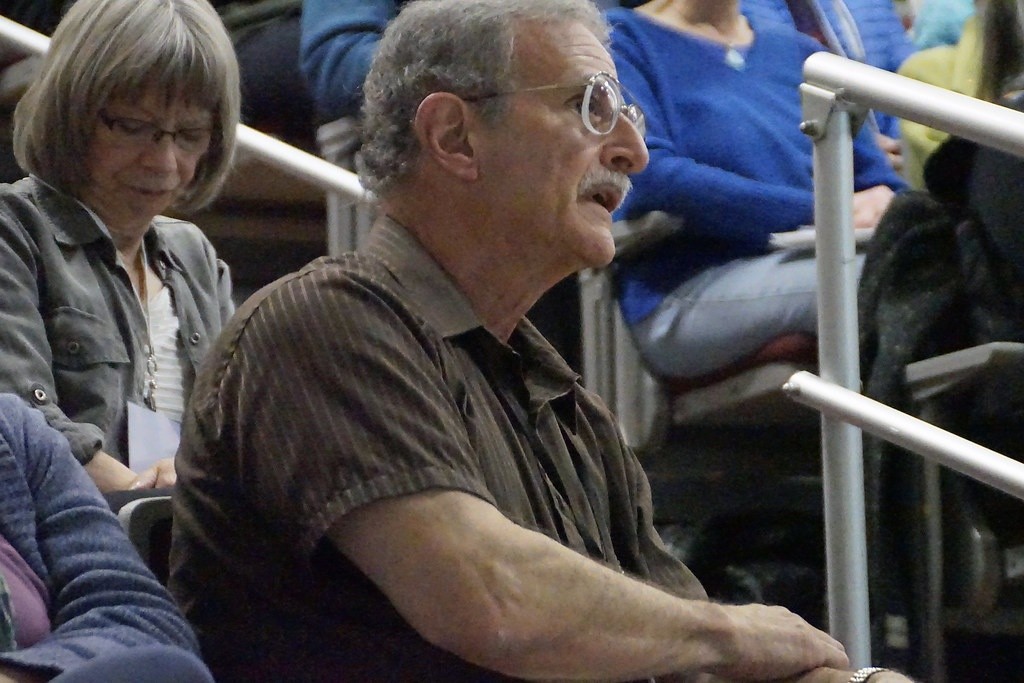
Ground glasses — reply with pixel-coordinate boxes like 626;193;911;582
98;108;222;154
397;71;646;142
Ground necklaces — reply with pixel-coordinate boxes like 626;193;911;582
698;19;749;69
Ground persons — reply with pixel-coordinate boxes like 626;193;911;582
169;1;1024;683
2;385;214;682
0;2;246;579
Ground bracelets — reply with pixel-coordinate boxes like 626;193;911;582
849;664;888;682
